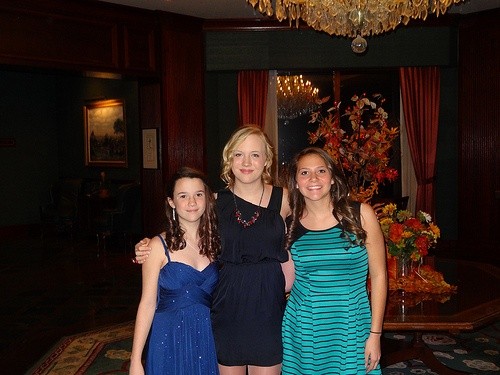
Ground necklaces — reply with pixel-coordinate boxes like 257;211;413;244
183;235;200;255
233;184;264;228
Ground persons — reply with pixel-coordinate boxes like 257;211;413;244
129;167;220;375
280;147;389;375
135;125;380;375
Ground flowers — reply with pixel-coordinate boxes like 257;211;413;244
380;204;440;264
307;89;402;207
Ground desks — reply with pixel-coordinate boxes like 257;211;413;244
380;290;500;331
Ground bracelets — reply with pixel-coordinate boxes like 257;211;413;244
370;330;382;335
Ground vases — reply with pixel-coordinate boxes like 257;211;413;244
397;259;411;296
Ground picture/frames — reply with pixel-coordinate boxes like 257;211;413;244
82;98;129;169
